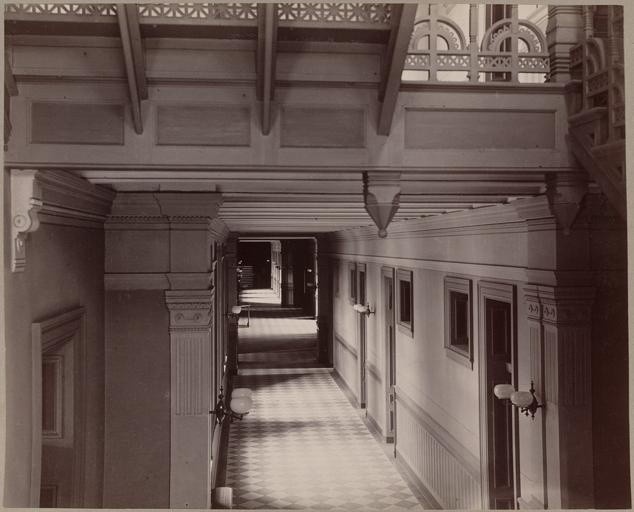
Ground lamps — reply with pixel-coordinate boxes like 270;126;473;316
493;379;545;421
209;384;254;426
353;303;375;317
225;306;242;321
